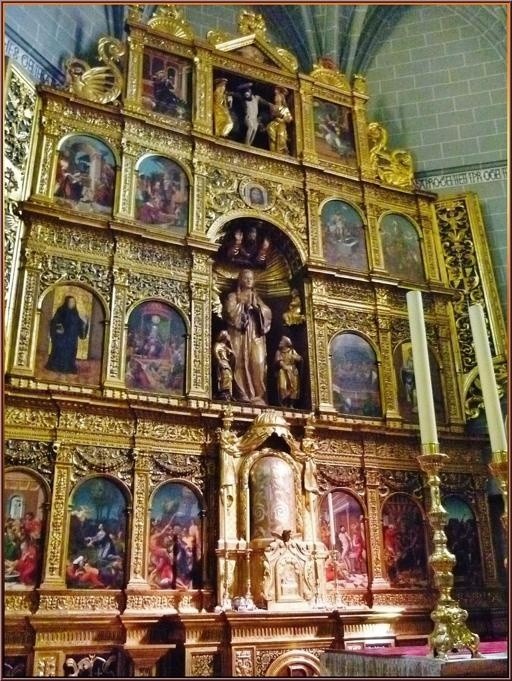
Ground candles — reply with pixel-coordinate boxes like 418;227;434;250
406;291;441;456
467;305;509;464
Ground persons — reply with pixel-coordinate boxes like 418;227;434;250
214;226;303;406
6;506;200;592
213;76;292;155
323;517;478;585
